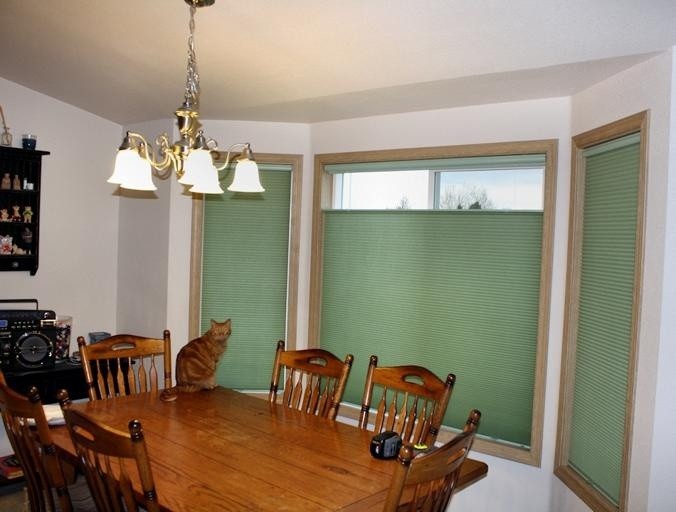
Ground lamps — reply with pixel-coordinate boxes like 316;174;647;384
105;0;265;197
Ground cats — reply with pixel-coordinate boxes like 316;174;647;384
159;318;232;401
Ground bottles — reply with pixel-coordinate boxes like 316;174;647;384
396;441;429;466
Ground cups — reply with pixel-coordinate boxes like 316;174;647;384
21;135;37;150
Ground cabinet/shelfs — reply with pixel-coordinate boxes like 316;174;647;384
0;147;50;276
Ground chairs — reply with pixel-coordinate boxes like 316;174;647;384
57;389;159;512
357;355;455;446
76;330;172;401
0;373;74;512
267;340;354;419
383;409;481;512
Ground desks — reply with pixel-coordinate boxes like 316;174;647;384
27;384;487;512
0;358;135;494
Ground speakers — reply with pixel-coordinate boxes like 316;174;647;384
0;299;55;373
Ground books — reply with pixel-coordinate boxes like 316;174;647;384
0;453;25;481
